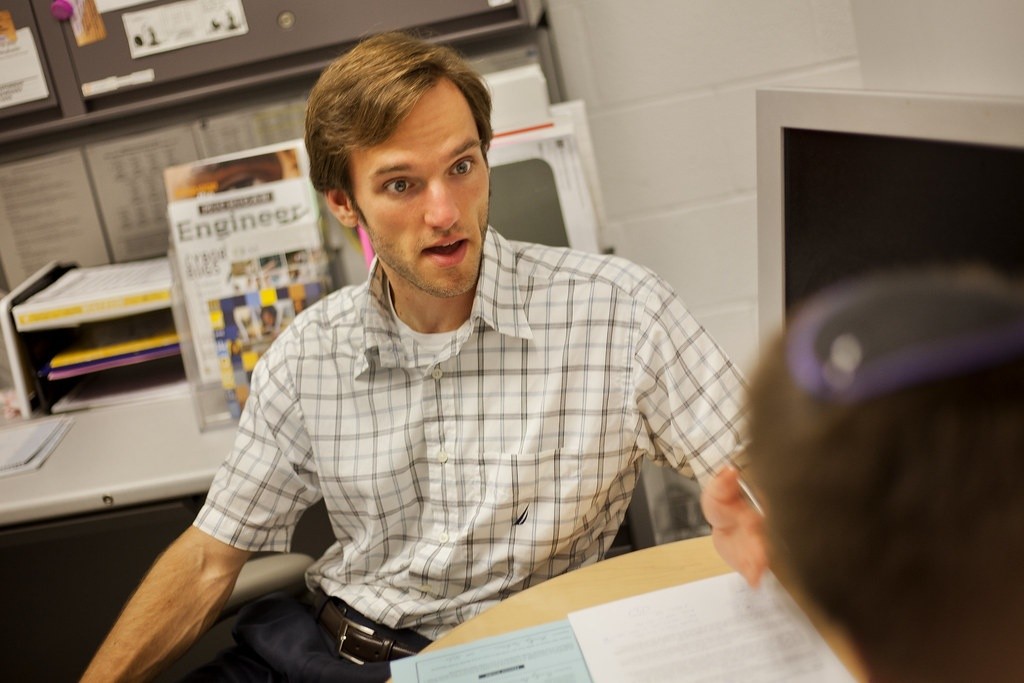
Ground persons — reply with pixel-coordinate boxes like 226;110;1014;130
743;270;1024;683
80;32;770;682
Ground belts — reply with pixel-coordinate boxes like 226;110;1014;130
304;587;420;665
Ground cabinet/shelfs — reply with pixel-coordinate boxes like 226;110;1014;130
0;387;671;682
0;0;567;146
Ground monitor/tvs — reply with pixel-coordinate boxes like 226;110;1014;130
755;86;1023;362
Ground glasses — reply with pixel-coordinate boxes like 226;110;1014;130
793;260;1024;411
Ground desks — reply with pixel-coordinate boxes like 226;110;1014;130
418;536;870;683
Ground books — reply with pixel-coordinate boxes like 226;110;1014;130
479;63;608;255
164;137;339;416
1;260;190;478
